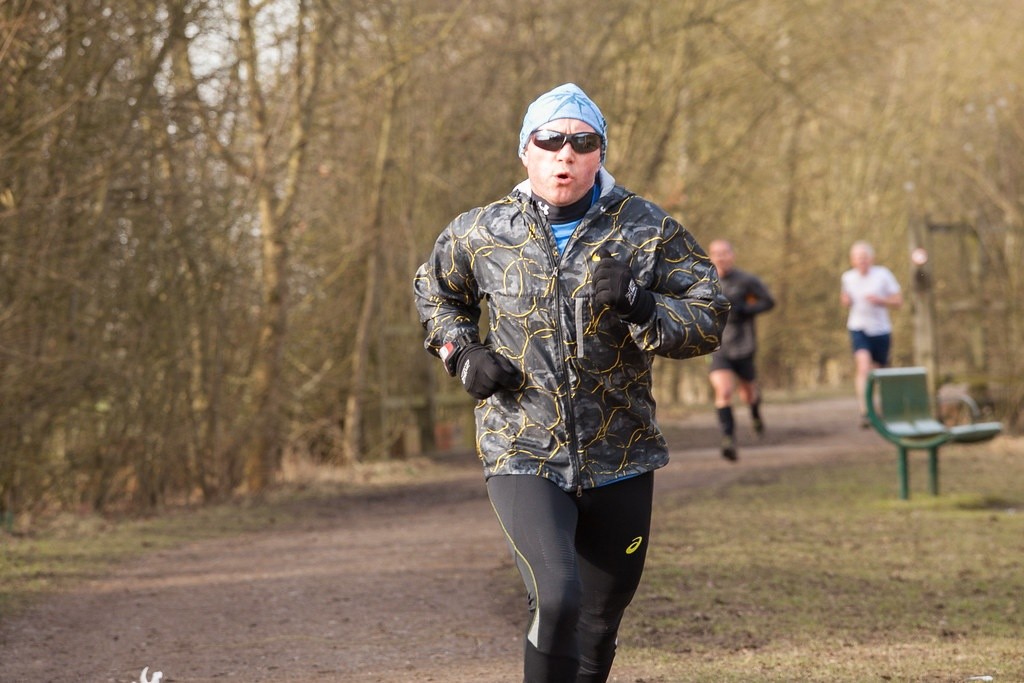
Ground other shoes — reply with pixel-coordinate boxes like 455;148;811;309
722;435;737;462
750;412;767;439
860;412;873;426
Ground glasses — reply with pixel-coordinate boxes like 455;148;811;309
530;128;602;154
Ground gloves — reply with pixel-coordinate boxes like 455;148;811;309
728;304;743;325
591;250;658;328
455;347;523;399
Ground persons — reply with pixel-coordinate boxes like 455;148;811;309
841;242;903;428
414;83;728;682
707;239;775;460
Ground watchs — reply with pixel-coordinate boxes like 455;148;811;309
438;333;478;376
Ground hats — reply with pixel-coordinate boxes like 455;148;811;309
518;82;607;170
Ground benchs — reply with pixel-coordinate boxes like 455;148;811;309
864;365;1005;500
